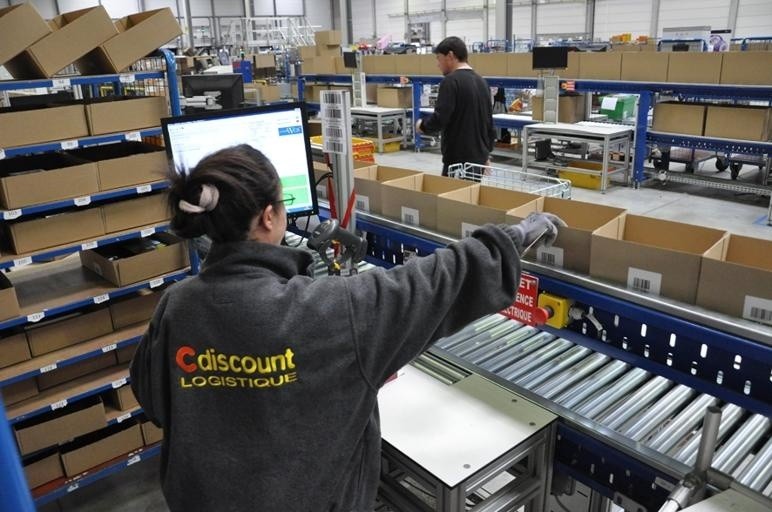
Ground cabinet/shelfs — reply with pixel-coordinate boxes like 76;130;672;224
0;2;199;512
296;71;771;204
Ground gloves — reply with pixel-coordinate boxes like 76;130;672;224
514;210;570;248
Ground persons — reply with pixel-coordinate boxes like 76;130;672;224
128;145;568;512
415;36;497;182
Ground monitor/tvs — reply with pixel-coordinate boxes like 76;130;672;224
161;100;319;245
343;52;357;68
181;72;245;114
533;46;568;78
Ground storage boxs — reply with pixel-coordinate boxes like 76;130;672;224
364;50;534;76
253;31;342;76
568;50;771;85
312;149;772;325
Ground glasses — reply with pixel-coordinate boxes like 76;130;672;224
273;193;296;206
